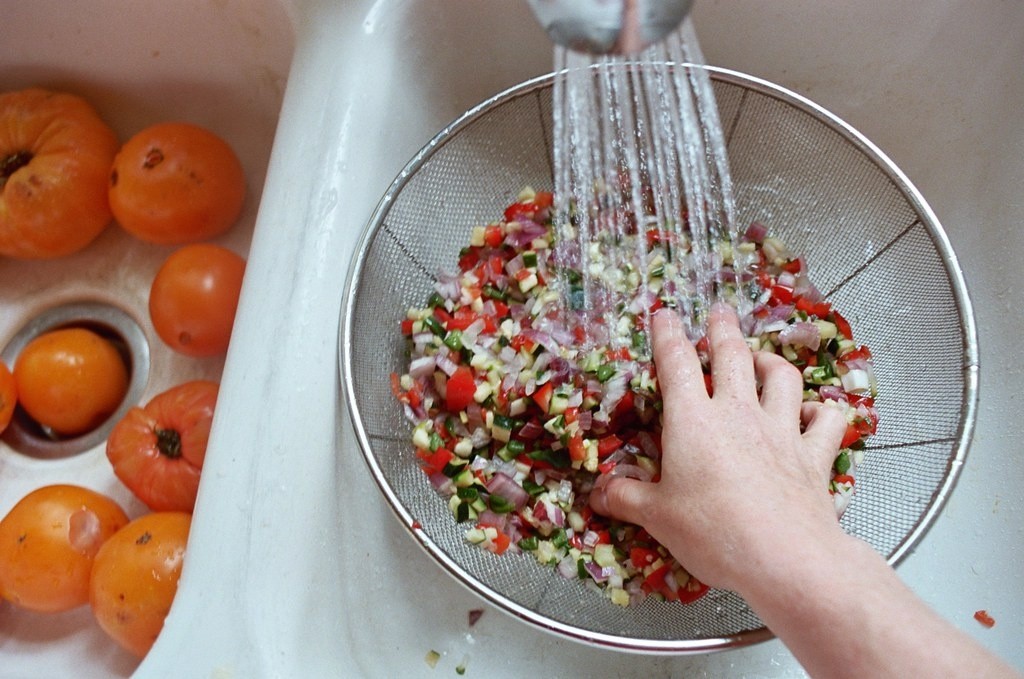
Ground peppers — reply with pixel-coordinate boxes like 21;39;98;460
398;191;876;605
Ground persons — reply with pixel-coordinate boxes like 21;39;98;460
589;301;1024;679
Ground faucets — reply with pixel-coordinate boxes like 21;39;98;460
528;2;695;57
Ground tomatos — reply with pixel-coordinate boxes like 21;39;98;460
1;86;246;659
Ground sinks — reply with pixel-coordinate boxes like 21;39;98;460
2;1;296;679
326;2;1024;679
